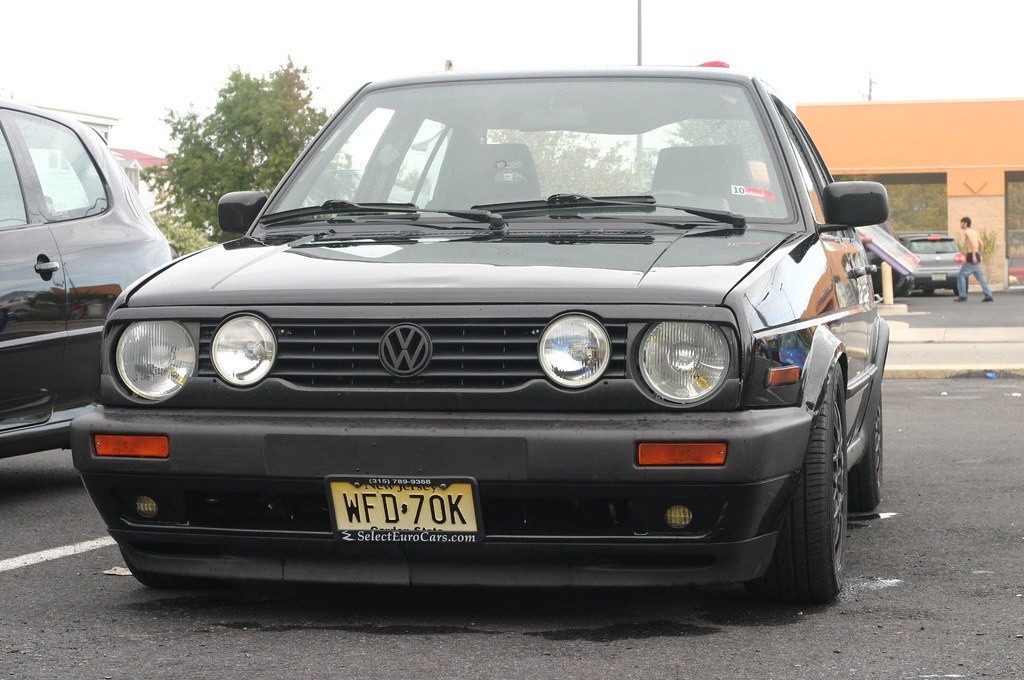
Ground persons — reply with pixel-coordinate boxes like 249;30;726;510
954;217;993;302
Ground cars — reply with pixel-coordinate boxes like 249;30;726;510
70;64;890;614
850;212;969;299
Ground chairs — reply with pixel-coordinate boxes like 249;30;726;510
427;140;543;212
650;141;753;208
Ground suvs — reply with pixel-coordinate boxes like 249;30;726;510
0;100;178;459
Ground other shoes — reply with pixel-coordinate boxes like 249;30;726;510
953;296;967;302
981;297;993;302
